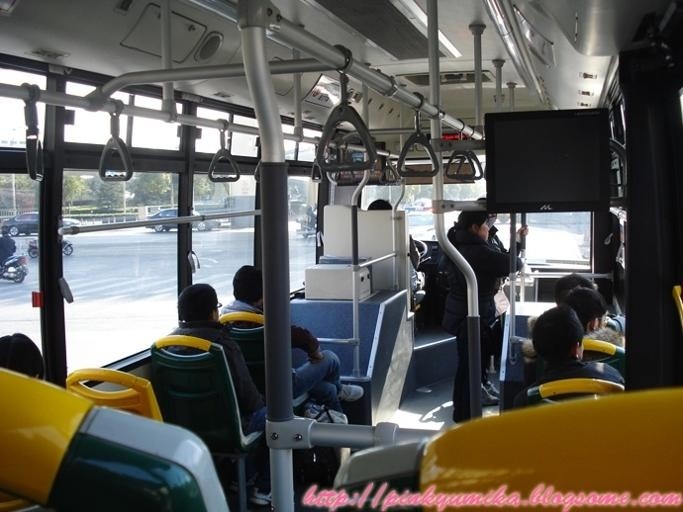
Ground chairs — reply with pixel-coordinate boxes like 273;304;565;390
331;386;683;512
147;335;265;511
66;367;161;420
0;368;231;511
510;376;626;406
216;312;309;407
582;339;624;376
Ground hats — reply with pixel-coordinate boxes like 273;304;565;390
233;266;263;301
177;285;218;322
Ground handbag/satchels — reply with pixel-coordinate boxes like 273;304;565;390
304;401;347;424
494;290;509;317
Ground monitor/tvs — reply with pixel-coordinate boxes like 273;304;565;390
485;107;610;213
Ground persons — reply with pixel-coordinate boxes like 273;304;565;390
367;199;425;298
0;230;17;267
507;304;624;410
448;212;522;424
508;287;626;377
473;197;526;405
162;283;271;505
219;265;363;423
543;275;610;303
0;332;46;380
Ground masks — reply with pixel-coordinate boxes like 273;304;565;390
486;216;496;231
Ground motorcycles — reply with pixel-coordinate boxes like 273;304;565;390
0;251;30;284
295;198;320;242
28;236;75;260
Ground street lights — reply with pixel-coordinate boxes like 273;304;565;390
4;120;24;219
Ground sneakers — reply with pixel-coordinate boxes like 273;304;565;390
487;382;499;397
338;384;363;402
248;487;271;505
480;384;500;405
230;471;259;494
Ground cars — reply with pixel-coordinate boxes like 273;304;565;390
400;205;441;243
0;209;83;236
144;206;215;236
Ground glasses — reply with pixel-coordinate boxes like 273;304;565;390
215;303;222;309
595;317;609;327
